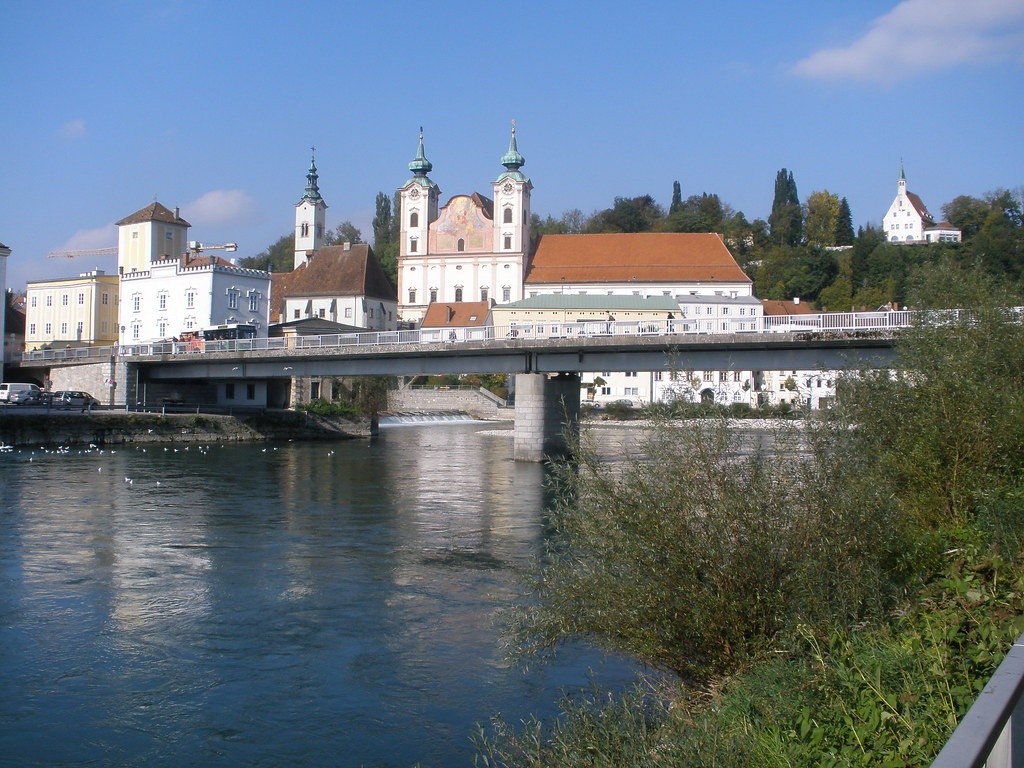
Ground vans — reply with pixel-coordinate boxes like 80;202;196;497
0;382;42;403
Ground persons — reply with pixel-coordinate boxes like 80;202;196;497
81;395;90;413
605;311;611;336
667;312;677;335
449;329;456;342
173;334;223;354
113;339;118;356
505;330;519;339
33;347;37;351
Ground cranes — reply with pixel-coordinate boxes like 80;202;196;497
47;241;238;263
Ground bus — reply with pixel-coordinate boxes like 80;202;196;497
204;324;257;351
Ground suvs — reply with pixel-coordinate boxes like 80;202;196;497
51;390;99;411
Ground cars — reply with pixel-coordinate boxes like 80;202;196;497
9;388;55;405
